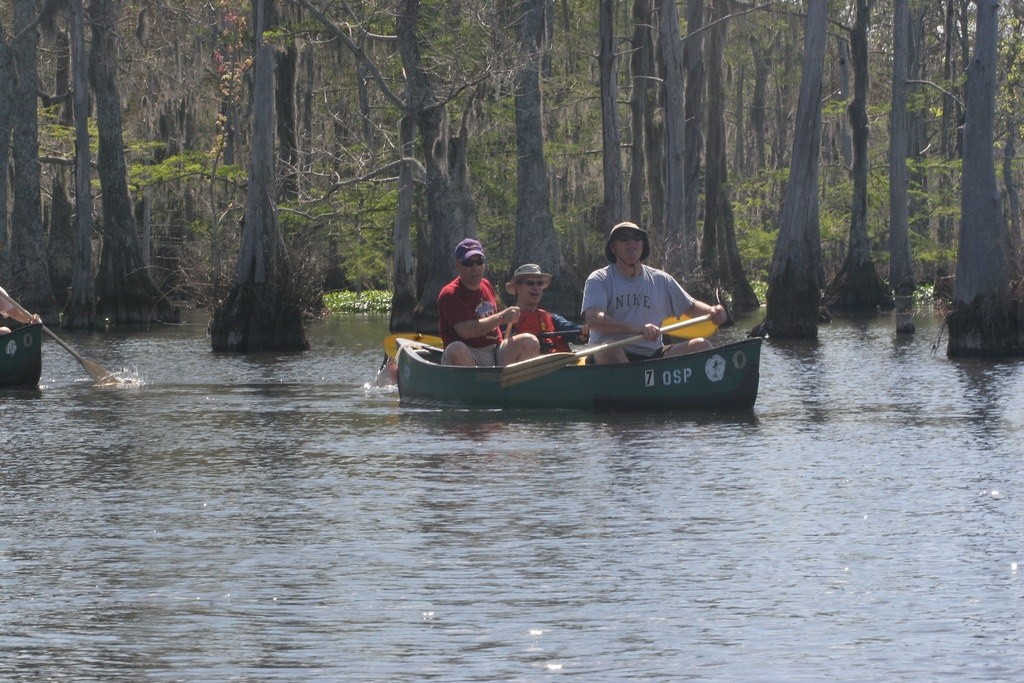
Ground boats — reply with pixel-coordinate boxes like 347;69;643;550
394;336;762;412
0;322;44;388
382;307;718;385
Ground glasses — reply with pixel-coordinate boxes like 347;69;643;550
618;234;642;242
462;258;484;266
525;280;544;286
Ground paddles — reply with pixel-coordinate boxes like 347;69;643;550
383;327;583;359
498;313;712;388
0;288;120;384
504;309;517;339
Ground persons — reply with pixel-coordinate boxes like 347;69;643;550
0;286;41;335
505;264;590;354
437;238;540;367
581;222;727;365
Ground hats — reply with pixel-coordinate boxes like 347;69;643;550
506;264;552;296
454;239;483;263
605;222;650;263
